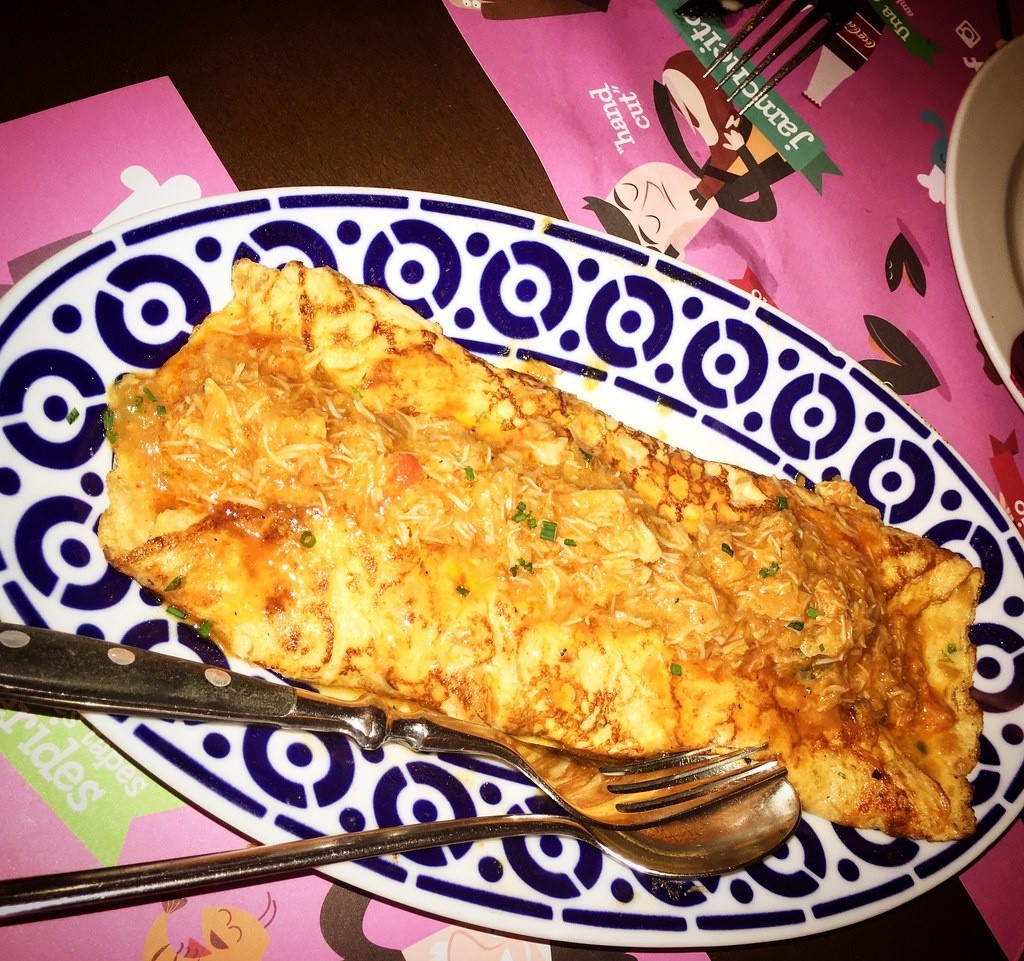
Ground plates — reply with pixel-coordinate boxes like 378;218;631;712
0;186;1024;951
942;34;1024;414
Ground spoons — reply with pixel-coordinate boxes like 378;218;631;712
0;777;800;931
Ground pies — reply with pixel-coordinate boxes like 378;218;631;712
95;257;985;842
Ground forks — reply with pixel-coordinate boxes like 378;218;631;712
701;0;862;118
0;622;789;829
